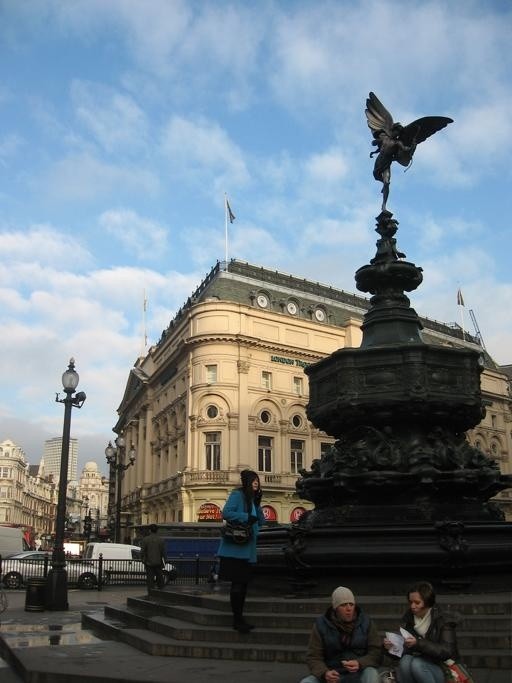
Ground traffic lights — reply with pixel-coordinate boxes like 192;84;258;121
83;524;89;536
85;516;89;522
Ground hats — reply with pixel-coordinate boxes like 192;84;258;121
241;470;257;487
332;586;355;610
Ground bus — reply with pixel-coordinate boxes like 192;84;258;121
131;523;290;578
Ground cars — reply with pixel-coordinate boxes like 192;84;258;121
1;531;107;590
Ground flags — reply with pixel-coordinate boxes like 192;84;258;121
226;196;236;224
457;287;465;308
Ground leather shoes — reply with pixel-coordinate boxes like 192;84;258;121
233;616;256;634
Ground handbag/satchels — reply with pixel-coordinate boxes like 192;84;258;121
221;525;253;544
439;659;477;683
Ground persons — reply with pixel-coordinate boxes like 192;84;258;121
384;582;460;683
216;470;265;635
141;524;168;595
370;122;417;211
299;586;382;683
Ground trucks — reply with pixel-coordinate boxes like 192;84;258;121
0;522;39;558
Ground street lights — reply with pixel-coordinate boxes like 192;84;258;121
105;436;136;543
48;355;86;612
101;476;112;514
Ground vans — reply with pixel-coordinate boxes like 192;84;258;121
83;542;177;585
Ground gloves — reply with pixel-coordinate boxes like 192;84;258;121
249;515;257;524
